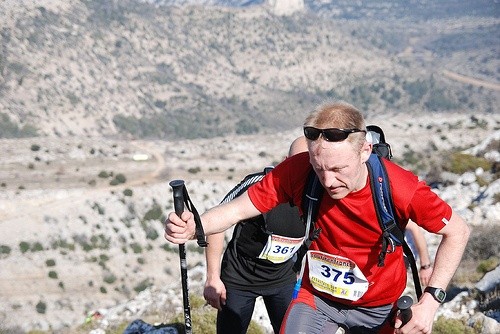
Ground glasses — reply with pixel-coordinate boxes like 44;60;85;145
304;127;371;141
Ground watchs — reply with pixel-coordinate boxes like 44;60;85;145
420;263;432;271
423;286;447;304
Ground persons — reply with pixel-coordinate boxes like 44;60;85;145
162;96;472;334
201;131;314;333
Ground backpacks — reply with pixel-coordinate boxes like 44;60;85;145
298;125;424;304
